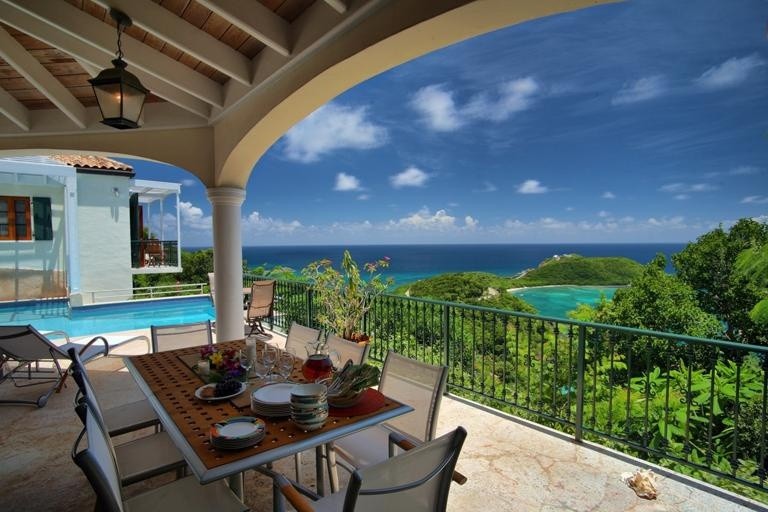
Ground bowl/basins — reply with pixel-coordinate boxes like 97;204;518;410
314;372;368;407
290;383;329;432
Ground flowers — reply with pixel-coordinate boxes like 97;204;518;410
197;343;250;379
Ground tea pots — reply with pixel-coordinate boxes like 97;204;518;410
301;341;342;382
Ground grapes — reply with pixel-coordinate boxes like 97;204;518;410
214;375;241;398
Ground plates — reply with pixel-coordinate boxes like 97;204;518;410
194;381;247;402
209;416;266;449
250;381;295;419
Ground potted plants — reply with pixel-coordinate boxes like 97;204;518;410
299;246;393;362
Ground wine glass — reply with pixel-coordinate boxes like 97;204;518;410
238;340;297;383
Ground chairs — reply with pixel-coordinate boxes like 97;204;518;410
244;279;279;339
283;319;325;361
270;425;467;511
330;350;452;473
68;402;249;511
317;333;371;374
149;318;213;355
0;324;110;407
67;345;165;450
205;272;216;330
67;369;192;487
147;237;164;265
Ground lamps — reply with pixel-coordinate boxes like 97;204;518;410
86;7;152;130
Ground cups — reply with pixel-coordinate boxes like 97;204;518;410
245;337;257;359
197;359;210;375
